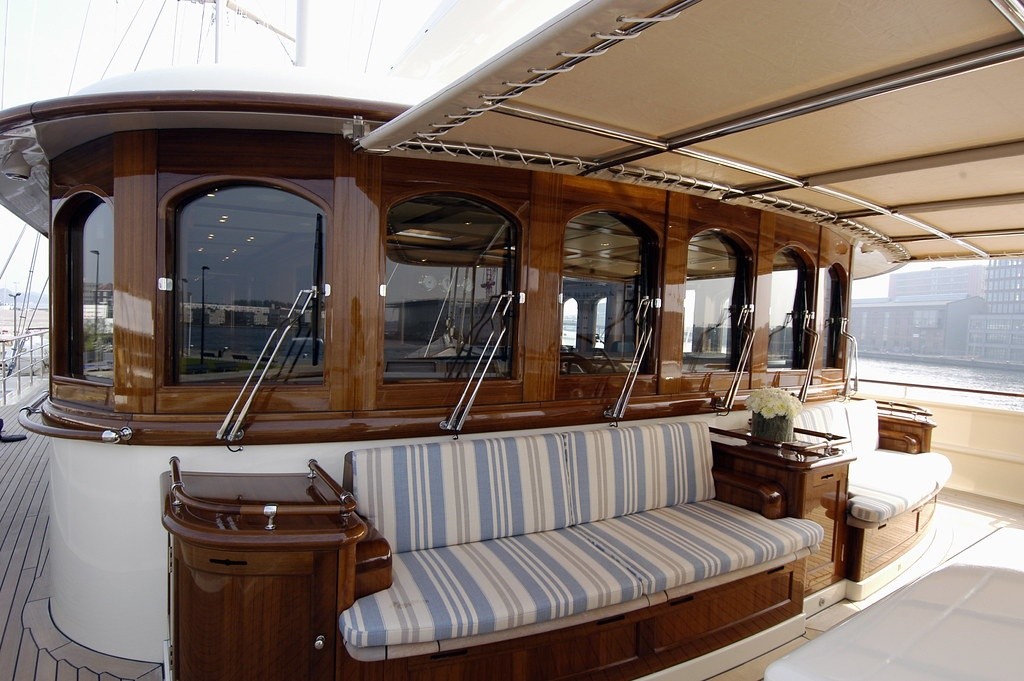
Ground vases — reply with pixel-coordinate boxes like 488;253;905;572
751;411;794;442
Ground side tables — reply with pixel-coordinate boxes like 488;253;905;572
851;397;937;453
709;419;857;600
158;456;368;681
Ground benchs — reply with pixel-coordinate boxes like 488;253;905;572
339;421;825;681
795;398;951;601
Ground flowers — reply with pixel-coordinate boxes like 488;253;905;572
744;386;804;420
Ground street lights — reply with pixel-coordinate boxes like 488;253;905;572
181;277;188;357
201;266;210;365
9;293;21;337
90;250;99;338
